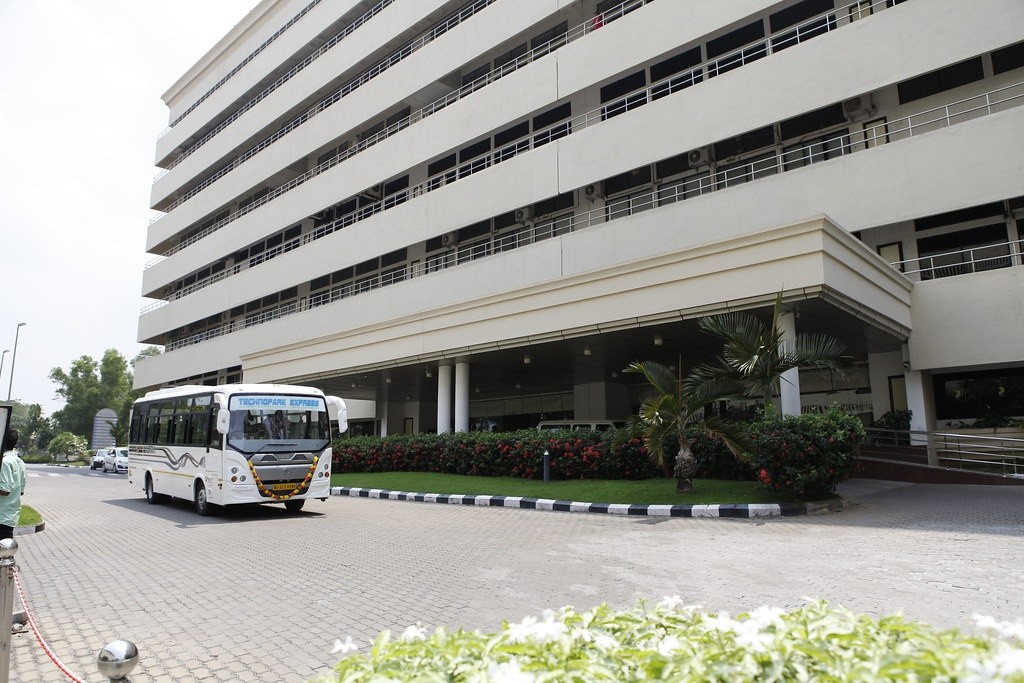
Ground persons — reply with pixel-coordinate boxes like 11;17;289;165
473;416;496;432
0;429;27;541
194;331;200;343
265;410;287;439
592;12;603;30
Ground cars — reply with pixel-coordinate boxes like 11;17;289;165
102;447;128;474
90;449;108;470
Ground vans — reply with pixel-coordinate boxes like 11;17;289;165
537;418;627;433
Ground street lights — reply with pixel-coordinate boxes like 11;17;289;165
0;349;10;380
7;323;27;401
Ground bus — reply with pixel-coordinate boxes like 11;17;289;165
127;384;348;517
